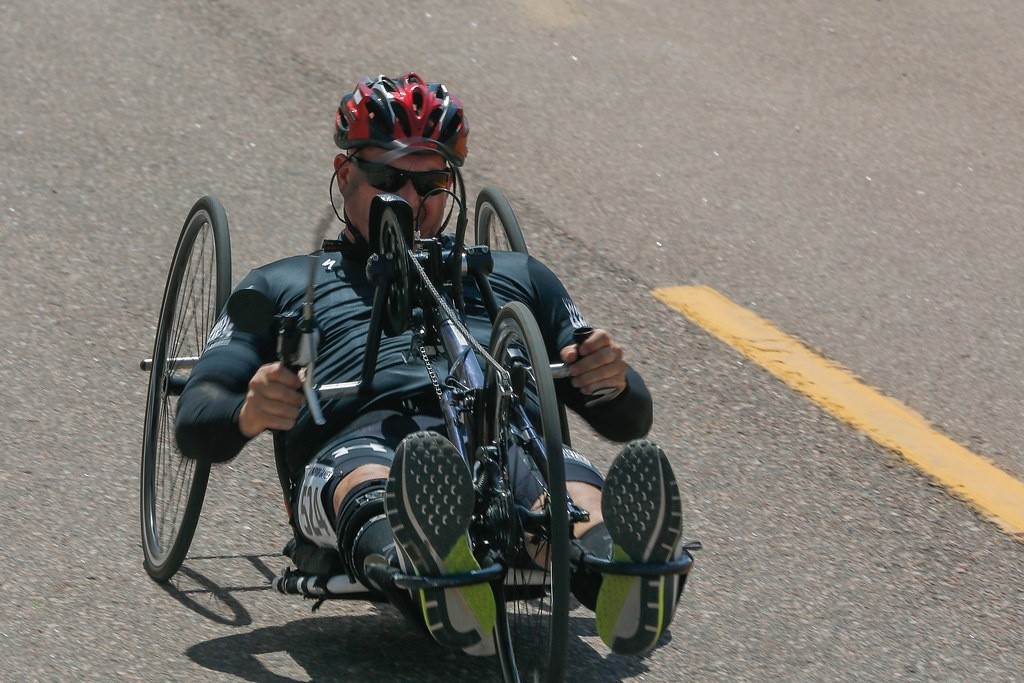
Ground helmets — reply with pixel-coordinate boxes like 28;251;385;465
332;71;469;167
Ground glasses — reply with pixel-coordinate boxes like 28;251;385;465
347;154;453;196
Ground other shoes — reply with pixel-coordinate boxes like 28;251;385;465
384;431;496;649
596;440;684;655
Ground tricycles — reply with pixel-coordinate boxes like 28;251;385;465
140;188;702;683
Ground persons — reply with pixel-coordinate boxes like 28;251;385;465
175;71;684;657
